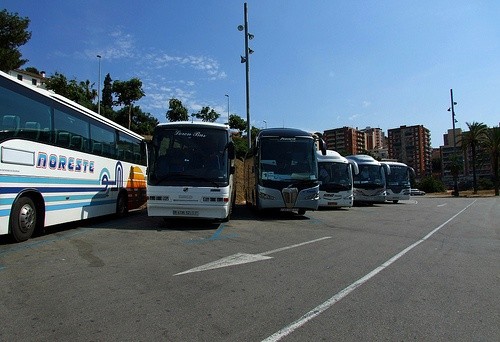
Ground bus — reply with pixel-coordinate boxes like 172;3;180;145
253;128;327;216
0;71;150;243
317;149;359;210
345;154;391;207
146;117;237;223
380;161;416;204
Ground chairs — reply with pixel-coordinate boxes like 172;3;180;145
0;115;141;163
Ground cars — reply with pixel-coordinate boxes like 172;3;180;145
411;189;426;196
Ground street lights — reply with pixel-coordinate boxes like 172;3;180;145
447;88;458;190
238;3;254;149
96;54;101;115
225;94;230;125
